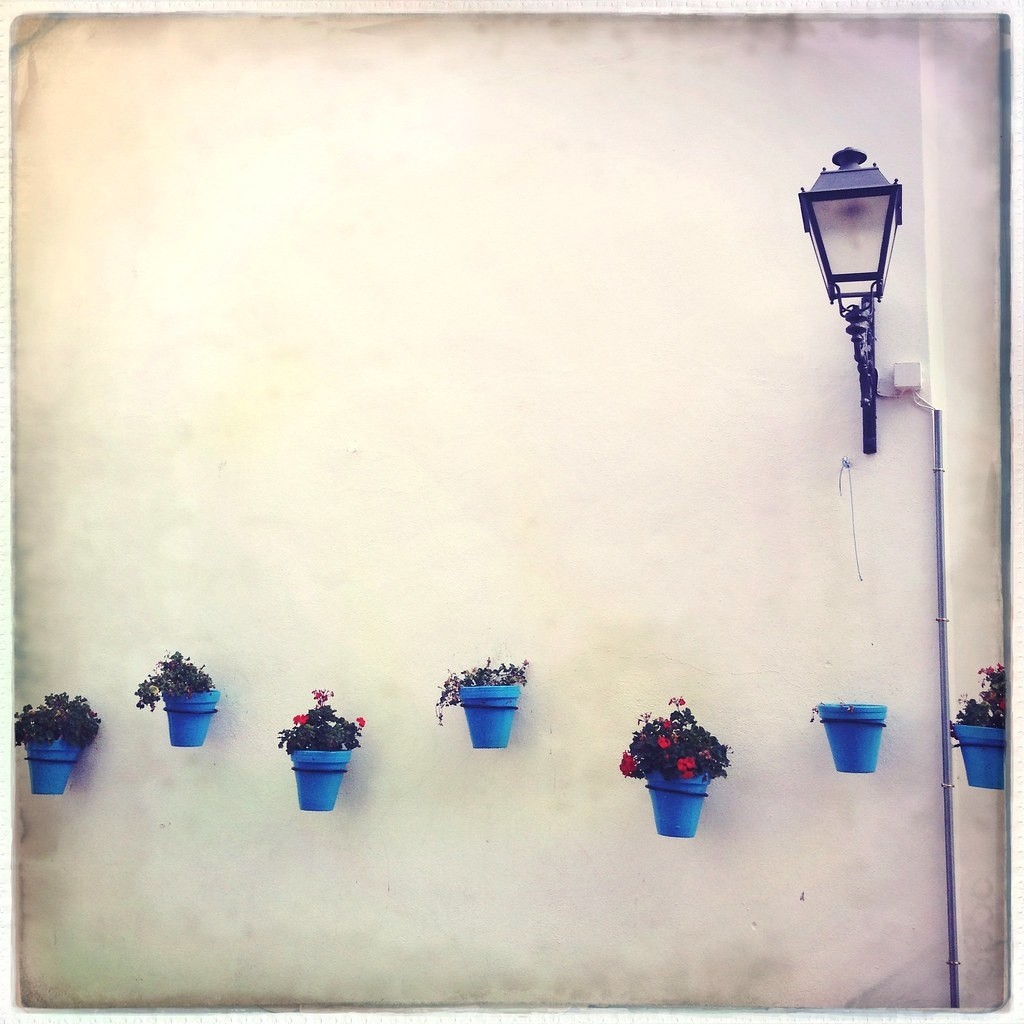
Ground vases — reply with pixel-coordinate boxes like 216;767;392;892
24;739;83;795
290;751;353;812
643;770;710;837
810;702;887;774
160;690;220;748
953;724;1005;790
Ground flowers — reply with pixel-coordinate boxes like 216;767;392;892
618;696;734;781
276;690;366;754
134;649;216;712
14;692;102;747
949;663;1007;737
435;657;530;726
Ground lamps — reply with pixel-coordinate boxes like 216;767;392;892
796;147;902;456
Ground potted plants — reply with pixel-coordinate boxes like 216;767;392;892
459;684;521;749
